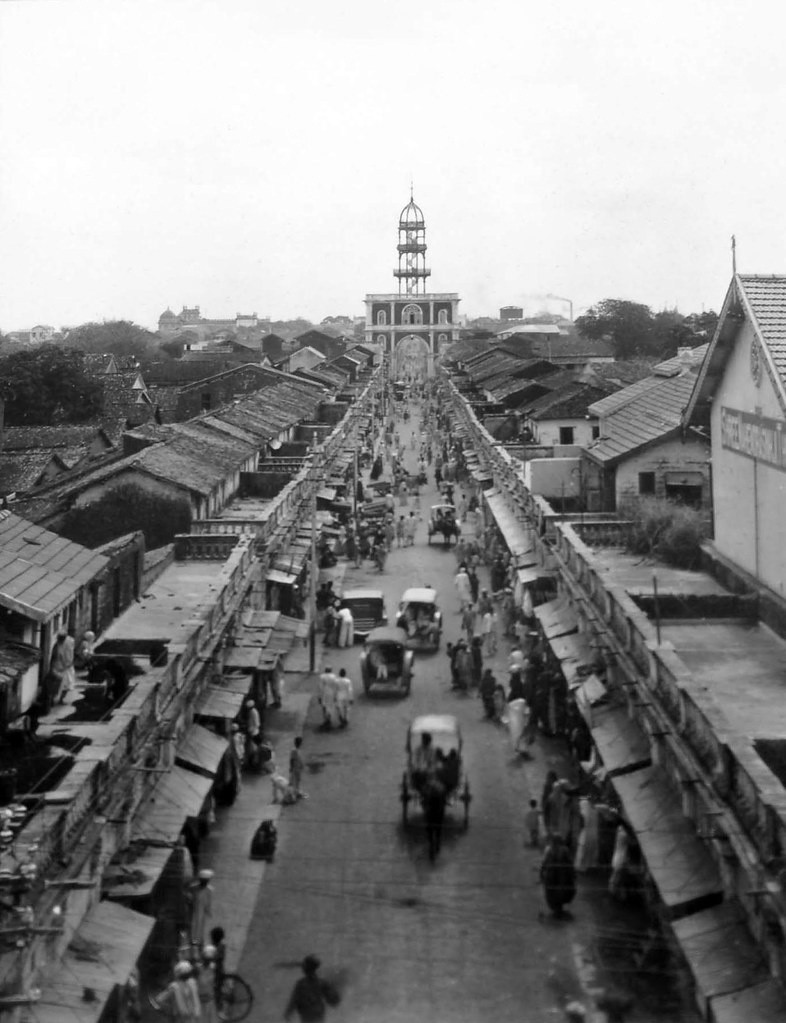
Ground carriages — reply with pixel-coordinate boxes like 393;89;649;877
396;583;445;649
428;505;460;547
400;713;473;867
360;615;414;698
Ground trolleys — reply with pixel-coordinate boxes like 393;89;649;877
375;485;397;495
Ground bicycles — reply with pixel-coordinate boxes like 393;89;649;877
146;941;254;1022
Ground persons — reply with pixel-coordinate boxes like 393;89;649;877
47;358;654;1023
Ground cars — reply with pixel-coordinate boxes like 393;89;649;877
336;590;388;636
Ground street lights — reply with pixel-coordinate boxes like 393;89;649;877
546;294;573;321
